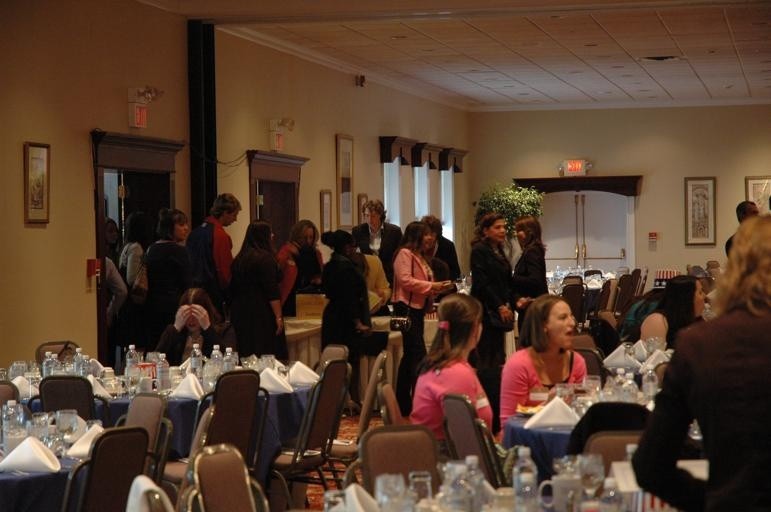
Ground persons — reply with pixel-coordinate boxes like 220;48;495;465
631;213;771;512
725;200;758;257
640;274;705;389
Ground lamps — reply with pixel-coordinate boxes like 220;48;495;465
130;85;164;103
266;115;298;134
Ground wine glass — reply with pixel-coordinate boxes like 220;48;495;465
553;453;606;503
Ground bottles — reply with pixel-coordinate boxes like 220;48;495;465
2;398;20;436
546;263;594;289
616;363;658;399
597;476;628;512
42;341;238;395
513;443;538;510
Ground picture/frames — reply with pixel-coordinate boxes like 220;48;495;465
320;190;332;244
358;193;369;226
24;142;52;226
683;176;717;246
744;175;770;218
335;133;354;231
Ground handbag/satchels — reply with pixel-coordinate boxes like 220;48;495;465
390;316;411;333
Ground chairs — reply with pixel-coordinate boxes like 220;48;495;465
1;266;728;510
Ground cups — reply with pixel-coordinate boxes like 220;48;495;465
0;357;41;398
85;419;103;433
555;371;602;413
625;444;638;459
30;411;66;457
375;456;488;511
241;352;277;373
536;475;581;512
